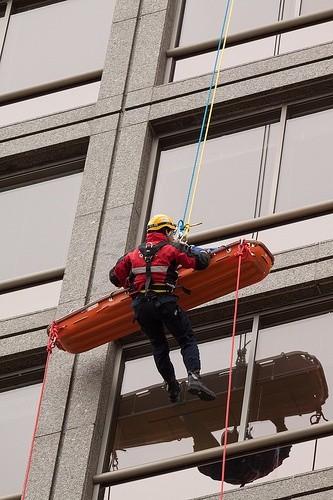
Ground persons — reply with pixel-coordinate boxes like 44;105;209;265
191;416;294;486
108;214;218;402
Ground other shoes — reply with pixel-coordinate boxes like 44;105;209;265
168;379;185;403
187;374;216;401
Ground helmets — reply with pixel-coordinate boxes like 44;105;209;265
147;214;177;232
